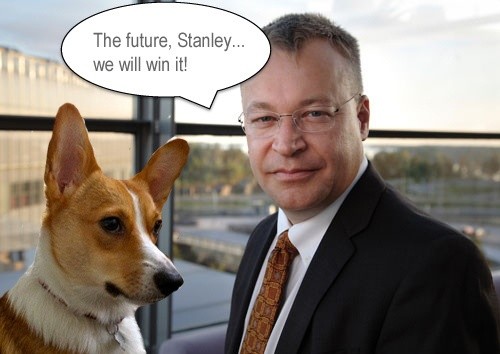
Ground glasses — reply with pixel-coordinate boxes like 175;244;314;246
237;88;364;138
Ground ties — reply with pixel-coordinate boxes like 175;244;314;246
240;229;300;354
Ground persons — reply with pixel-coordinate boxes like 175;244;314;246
223;11;499;354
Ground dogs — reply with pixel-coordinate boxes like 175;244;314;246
0;102;191;350
